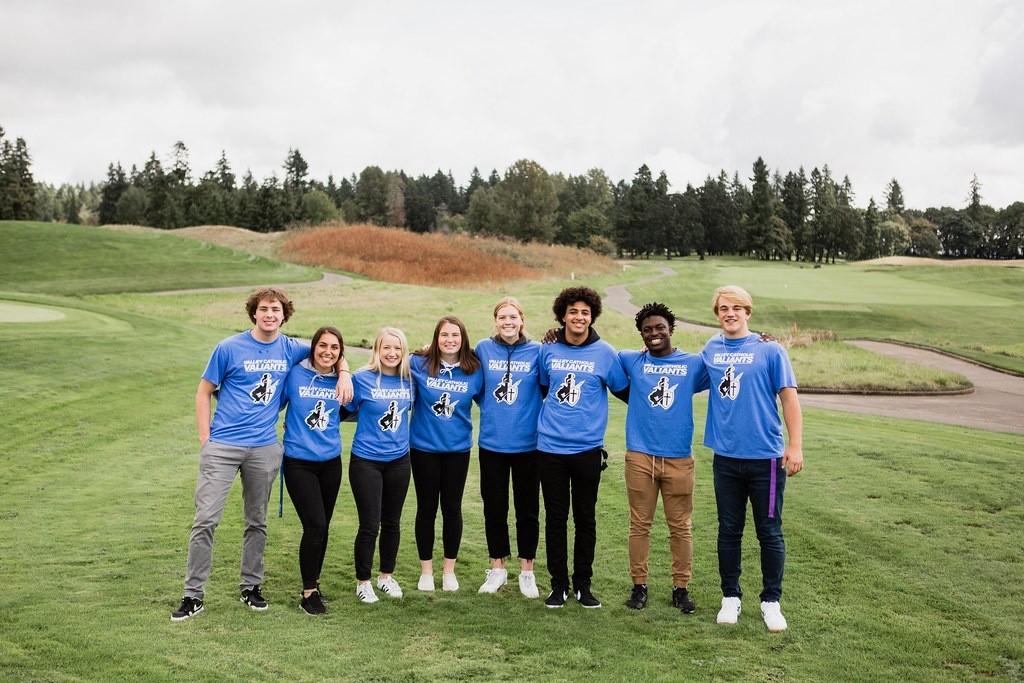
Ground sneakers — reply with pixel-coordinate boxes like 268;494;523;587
715;597;741;624
376;576;403;598
418;575;434;591
761;601;787;631
572;584;602;608
170;596;205;621
623;584;648;611
478;568;508;593
356;580;379;603
519;574;540;598
299;588;329;616
672;586;696;614
240;585;269;611
543;584;571;607
443;573;460;592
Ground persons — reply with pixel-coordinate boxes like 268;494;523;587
283;326;415;603
212;326;343;617
407;294;543;599
536;285;803;633
170;286;354;621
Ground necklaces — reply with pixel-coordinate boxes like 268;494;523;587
722;333;751;367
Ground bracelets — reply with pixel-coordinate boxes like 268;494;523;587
339;370;351;376
